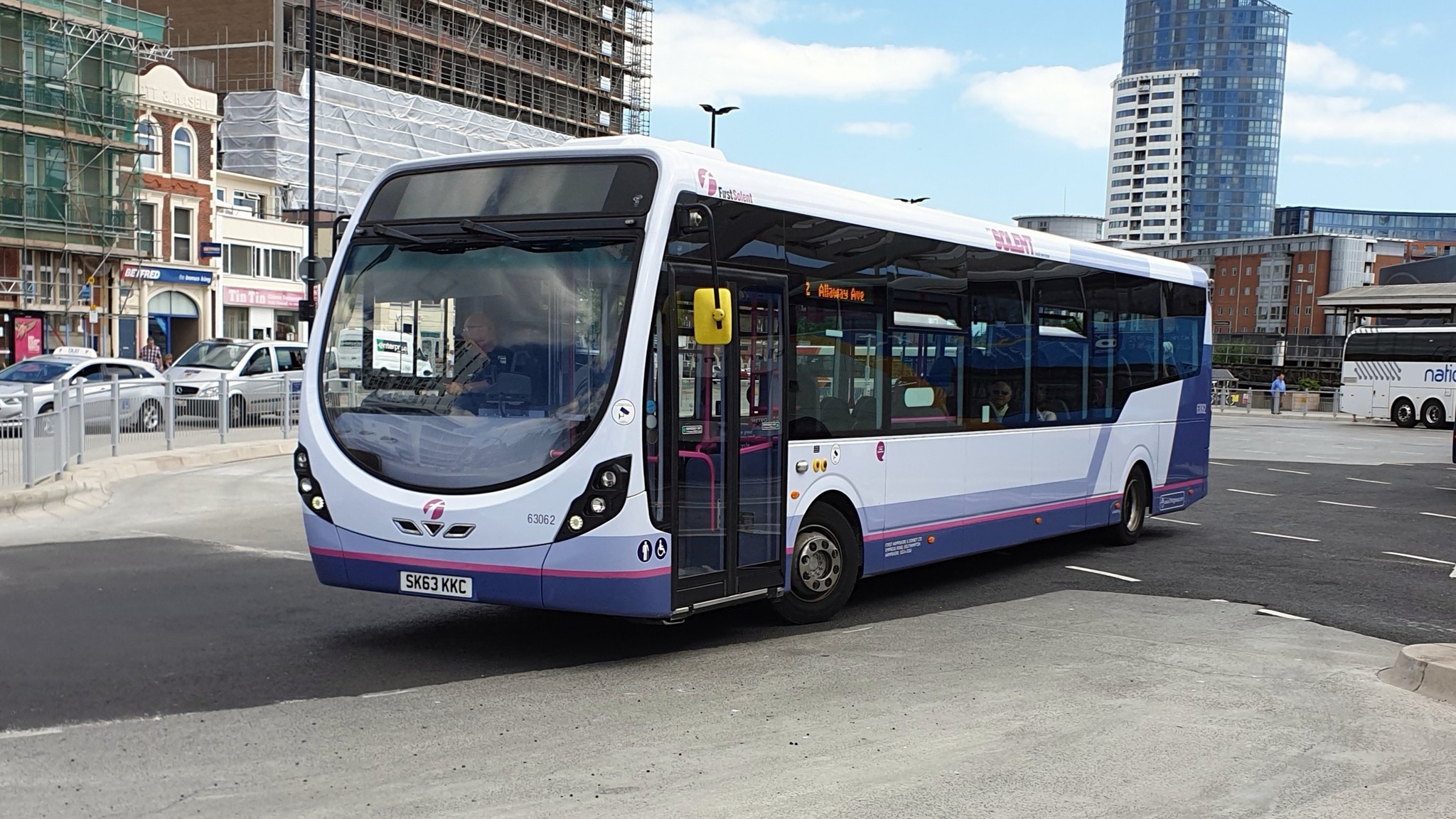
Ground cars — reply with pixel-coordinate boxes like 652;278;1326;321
0;346;169;437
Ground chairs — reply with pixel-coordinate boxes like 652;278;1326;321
821;341;1183;436
290;352;301;366
249;350;261;366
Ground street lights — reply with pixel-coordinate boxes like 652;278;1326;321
334;153;352;251
1281;252;1295;374
699;104;741;148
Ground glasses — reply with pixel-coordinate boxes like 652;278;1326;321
993;390;1010;396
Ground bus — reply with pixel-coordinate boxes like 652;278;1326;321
291;132;1214;627
772;344;987;408
1337;325;1456;430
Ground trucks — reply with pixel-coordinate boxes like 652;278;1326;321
335;325;439;390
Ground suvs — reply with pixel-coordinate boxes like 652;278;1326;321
161;337;341;428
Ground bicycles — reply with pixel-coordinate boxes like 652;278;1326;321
1211;381;1235;406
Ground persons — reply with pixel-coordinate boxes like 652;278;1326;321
138;337;174;374
443;312;530;419
1271;374;1287;415
1037;407;1057;422
971;379;1023;428
